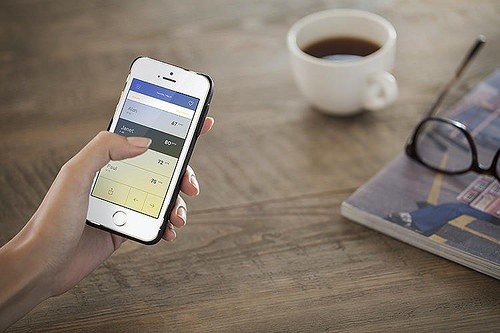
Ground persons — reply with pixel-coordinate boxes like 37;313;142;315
0;114;214;331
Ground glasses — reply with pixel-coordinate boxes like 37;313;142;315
402;32;499;185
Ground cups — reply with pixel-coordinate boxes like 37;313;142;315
286;9;397;116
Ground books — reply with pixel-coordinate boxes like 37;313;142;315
340;68;500;280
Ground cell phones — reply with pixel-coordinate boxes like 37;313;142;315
85;56;216;246
